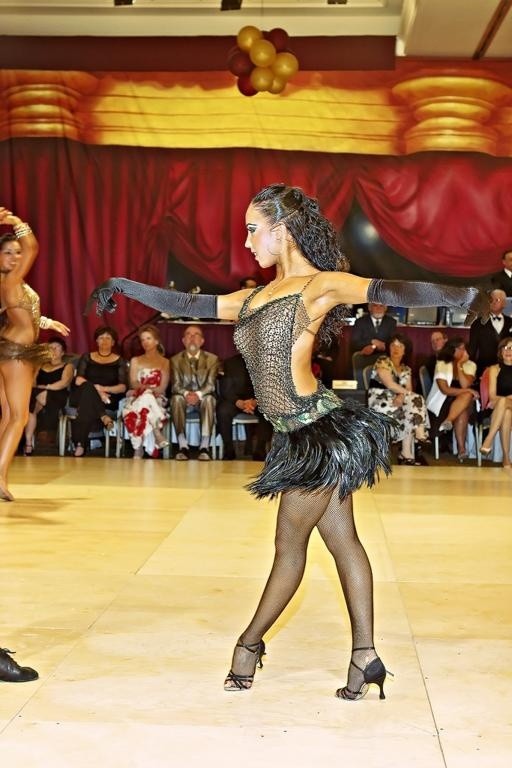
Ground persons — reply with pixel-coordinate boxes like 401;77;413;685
83;184;491;701
24;324;272;462
239;278;260;289
0;207;72;503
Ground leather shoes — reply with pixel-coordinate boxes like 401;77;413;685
154;441;171;450
198;448;211;461
253;451;267;460
223;451;237;459
175;447;189;460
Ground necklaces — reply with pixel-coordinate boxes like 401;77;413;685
266;273;298;297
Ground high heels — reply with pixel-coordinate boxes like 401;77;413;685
105;422;115;435
457;453;468;464
480;447;491;459
224;634;266;690
336;645;387;701
438;421;453;434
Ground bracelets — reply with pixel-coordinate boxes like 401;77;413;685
13;223;33;239
38;316;53;329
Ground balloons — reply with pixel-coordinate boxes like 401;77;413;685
226;24;302;99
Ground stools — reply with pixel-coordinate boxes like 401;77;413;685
24;393;507;465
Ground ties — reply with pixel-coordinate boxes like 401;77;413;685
374;319;380;333
189;357;199;391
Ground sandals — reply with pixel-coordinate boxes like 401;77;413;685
397;452;421;466
414;436;432;447
